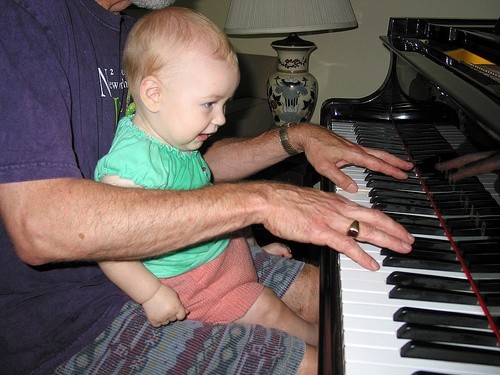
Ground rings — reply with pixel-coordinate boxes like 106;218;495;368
346;220;361;239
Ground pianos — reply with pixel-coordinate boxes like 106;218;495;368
320;18;500;375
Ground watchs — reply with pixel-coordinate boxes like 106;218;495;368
278;122;300;158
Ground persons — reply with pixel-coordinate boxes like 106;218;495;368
92;7;321;348
0;0;416;374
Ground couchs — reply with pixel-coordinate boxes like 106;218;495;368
197;52;280;156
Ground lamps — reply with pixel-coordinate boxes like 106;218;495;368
223;0;359;127
